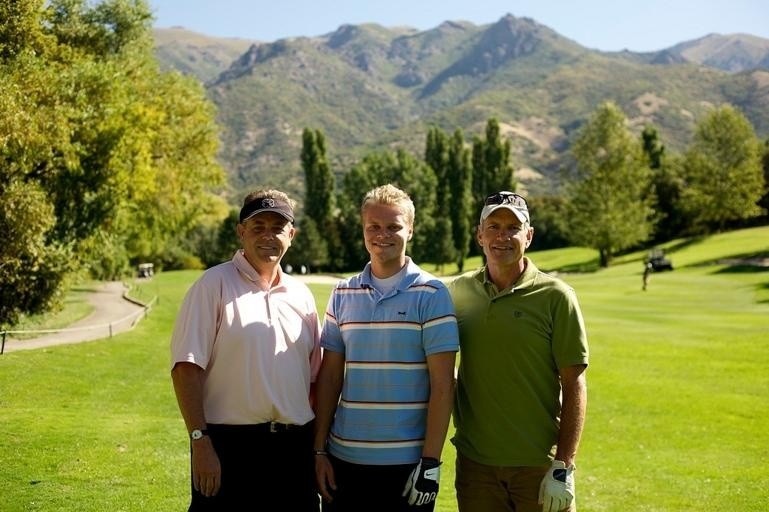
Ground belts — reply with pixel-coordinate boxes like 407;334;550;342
262;421;294;433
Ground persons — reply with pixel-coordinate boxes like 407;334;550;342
444;190;589;511
169;188;323;511
642;262;650;291
312;182;460;512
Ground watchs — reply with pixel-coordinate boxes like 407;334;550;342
190;428;208;441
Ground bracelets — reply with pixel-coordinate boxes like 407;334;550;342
313;450;328;456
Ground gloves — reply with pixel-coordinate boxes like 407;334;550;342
401;457;440;506
538;459;575;512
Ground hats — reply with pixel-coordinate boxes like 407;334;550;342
240;198;294;222
480;191;529;223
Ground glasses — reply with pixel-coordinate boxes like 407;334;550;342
486;193;528;207
